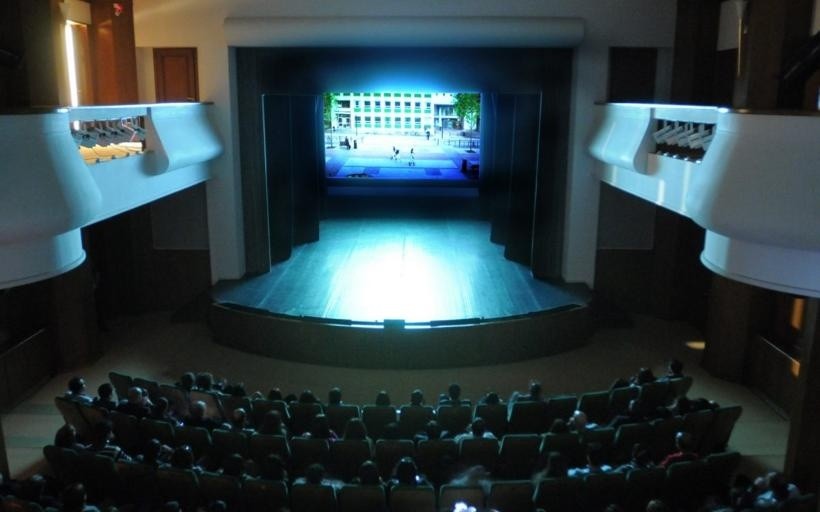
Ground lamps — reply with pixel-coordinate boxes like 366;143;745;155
651;119;716;161
69;115;147;162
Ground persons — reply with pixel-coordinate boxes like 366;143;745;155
426;127;432;142
391;146;400;158
2;357;803;510
408;144;417;166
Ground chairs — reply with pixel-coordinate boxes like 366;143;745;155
0;371;816;511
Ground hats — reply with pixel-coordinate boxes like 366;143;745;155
670;359;684;374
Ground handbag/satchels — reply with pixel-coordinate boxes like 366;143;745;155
480;453;527;480
